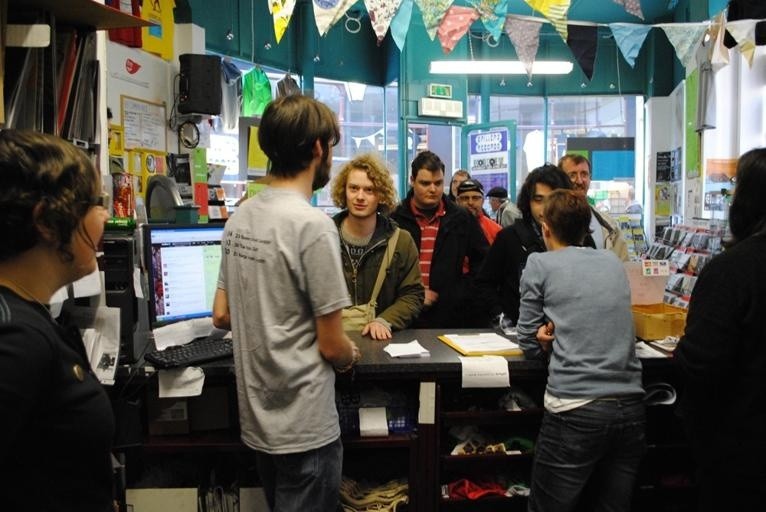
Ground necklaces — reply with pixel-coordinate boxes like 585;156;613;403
1;275;60;326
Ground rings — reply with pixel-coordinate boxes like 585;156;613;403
546;327;552;333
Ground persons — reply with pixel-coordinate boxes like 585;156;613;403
455;179;503;274
330;151;425;341
560;153;631;263
623;189;644;228
664;148;766;511
0;127;124;510
446;170;491;218
386;150;492;330
469;163;599;328
211;89;362;510
486;187;523;229
516;188;651;511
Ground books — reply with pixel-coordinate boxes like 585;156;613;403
614;214;721;312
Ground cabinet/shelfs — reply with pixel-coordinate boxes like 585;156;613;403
147;176;228;223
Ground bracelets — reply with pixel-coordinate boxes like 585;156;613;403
334;335;361;374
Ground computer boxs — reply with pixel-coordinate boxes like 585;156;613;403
97;229;149;364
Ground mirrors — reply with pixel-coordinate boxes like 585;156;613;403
180;121;201;148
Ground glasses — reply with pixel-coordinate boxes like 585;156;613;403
71;194;110;209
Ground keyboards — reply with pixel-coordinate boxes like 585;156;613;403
144;337;232;369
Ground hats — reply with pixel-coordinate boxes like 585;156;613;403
486;187;507;197
457;180;484;195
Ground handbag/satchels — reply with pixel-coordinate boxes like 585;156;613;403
341;303;375;331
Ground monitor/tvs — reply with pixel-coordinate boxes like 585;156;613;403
142;223;225;332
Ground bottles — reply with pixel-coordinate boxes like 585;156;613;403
133;197;148;227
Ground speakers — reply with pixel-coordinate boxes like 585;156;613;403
178;54;222;115
